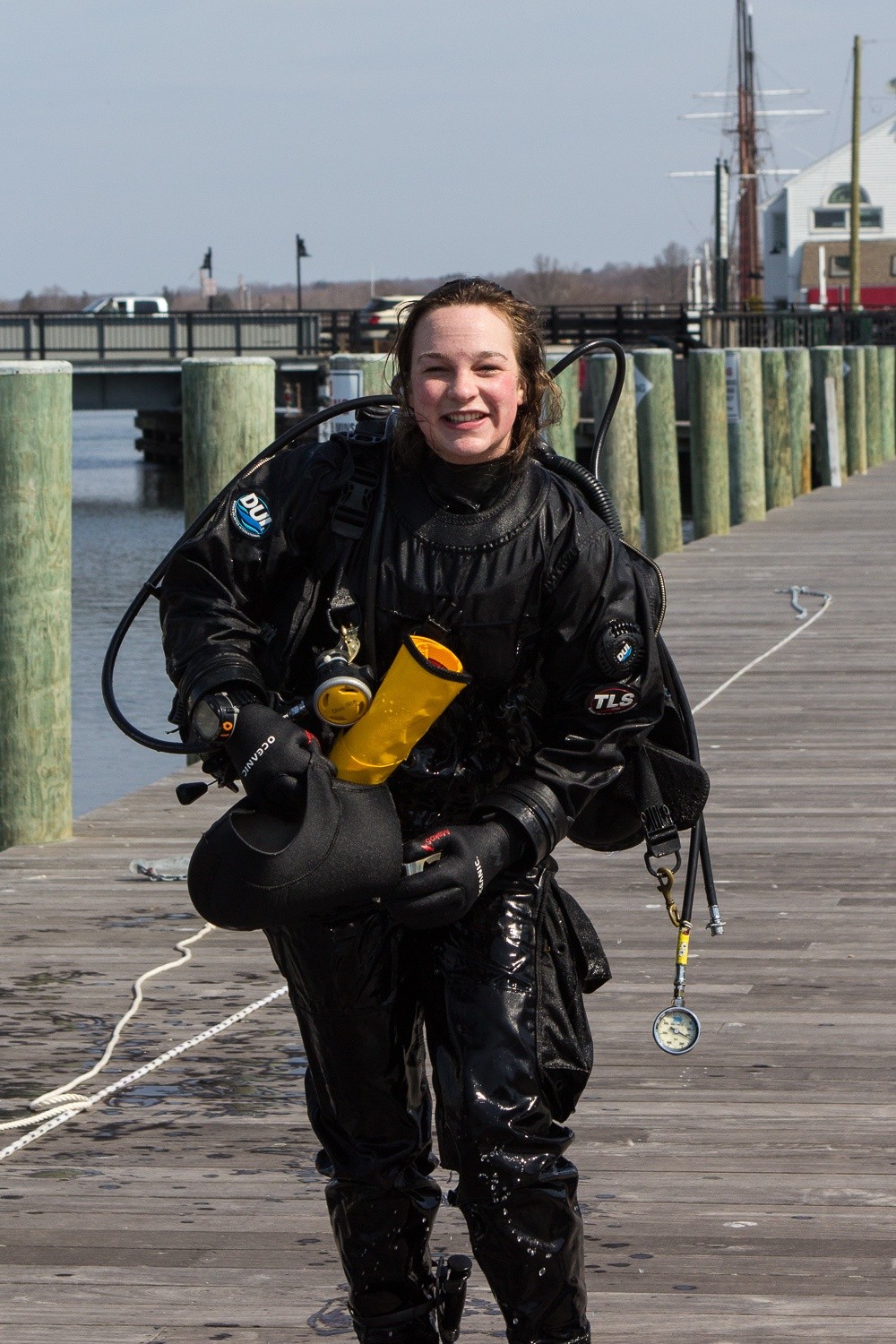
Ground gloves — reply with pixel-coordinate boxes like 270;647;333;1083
224;704;320;791
386;823;515;927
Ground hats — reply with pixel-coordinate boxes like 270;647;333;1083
188;761;402;933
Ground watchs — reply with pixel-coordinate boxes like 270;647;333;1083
190;686;259;748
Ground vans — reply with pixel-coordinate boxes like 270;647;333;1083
79;295;170;319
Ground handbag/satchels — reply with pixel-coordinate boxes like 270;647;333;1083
568;632;710;852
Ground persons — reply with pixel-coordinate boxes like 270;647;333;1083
158;275;664;1344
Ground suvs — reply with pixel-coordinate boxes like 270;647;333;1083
356;294;426;339
